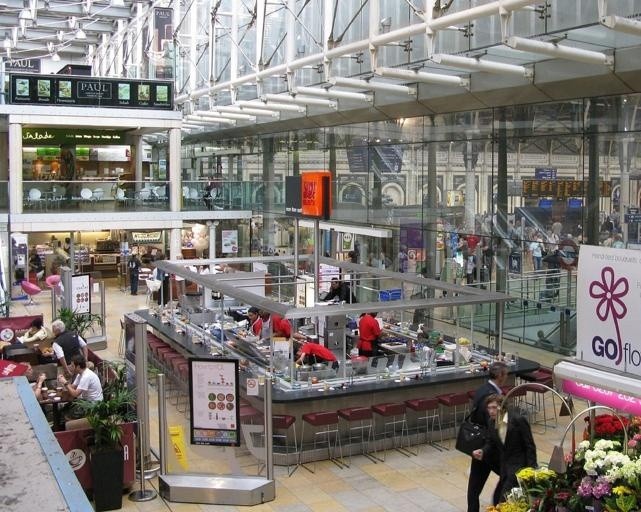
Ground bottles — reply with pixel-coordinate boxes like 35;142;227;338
455;361;460;374
506;351;520;361
308;377;313;392
431;364;436;377
376;370;381;385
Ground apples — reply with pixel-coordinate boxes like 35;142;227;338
312;377;318;384
480;361;488;367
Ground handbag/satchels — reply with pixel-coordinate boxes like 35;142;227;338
455;411;491;460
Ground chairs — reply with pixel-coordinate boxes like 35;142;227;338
26;186;221;209
22;274;61;306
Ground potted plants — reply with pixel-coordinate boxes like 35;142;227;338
73;365;141;511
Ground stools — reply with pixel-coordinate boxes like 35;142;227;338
147;331;557;474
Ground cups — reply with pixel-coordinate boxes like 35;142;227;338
211;346;217;355
33;343;40;352
41;387;48;399
258;375;265;386
55;388;63;398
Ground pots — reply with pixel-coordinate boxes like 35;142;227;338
298;356;325;372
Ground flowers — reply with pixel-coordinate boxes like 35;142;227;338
489;415;641;508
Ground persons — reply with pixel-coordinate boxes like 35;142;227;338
30;235;72;273
241;306;341;371
462;362;510;512
534;330;555;351
440;207;626;303
345;240;411;274
203;175;217;210
141;249;155;263
470;393;540;505
157;254;170;307
127;254;142;296
154;248;166;262
319;277;357;303
411;266;429;325
174;253;190;308
188;263;236;275
356;311;383;358
1;318;105;431
